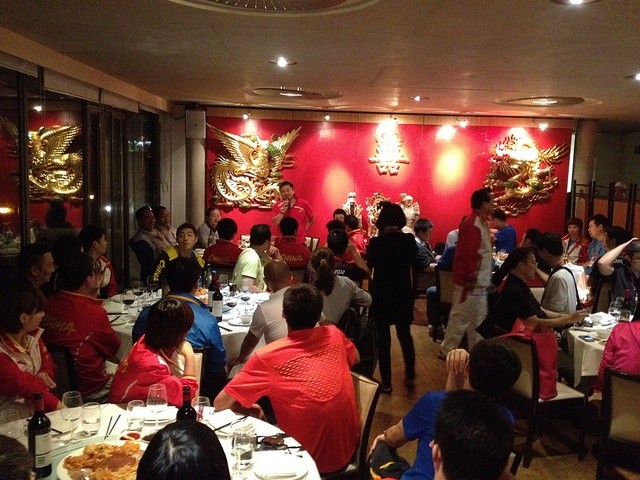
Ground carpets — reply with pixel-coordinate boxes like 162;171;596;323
412;294;430;326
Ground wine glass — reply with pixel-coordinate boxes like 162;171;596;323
616;297;624;301
219;274;228;288
240;286;250;314
191;396;211;425
133;281;144;308
237;234;250;249
147;275;159;299
146;384;168;431
608;301;622;325
227;429;251;480
60;391;83;444
0;409;23;439
124;289;135;318
225;291;239;319
120;292;133;314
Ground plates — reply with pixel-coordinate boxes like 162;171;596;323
254;454;308;480
107;315;128;325
57;440;149;480
110;294;138;303
144;407;179;423
217;321;234;332
589;314;615;326
207;416;255;437
588;332;610;342
575;327;608;331
26;415;78;435
229;318;253;326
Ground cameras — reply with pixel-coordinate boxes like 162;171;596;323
575;316;594;327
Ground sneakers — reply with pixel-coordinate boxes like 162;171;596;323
439;348;447;361
428;323;444;331
381;382;392;393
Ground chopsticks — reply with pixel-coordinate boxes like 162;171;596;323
218;326;233;332
213;415;250;431
110;316;121;323
27;419;62;434
104;415;121;440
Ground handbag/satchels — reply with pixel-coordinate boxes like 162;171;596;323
511;316;558;400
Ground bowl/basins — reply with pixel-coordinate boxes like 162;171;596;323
596;329;612;339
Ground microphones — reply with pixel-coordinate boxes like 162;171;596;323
281;197;292;210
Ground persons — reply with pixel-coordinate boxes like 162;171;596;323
365;337;522;480
492;208;516;253
334;209;346;222
1;285;70;424
364;204;419;395
0;435;36;480
201;218;243;265
535;232;590;342
584;226;627;313
343;215;371;256
271;181;315;247
152;222;208;298
136;420;231;480
227;259;326;379
444;216;469;251
41;249;124;403
519;228;550;273
128;206;171;282
78;225;118;298
274;217;312;268
414;218;442;288
107;298;199;410
582;214;613;274
327;229;370;283
596;237;640;314
152;207;178;247
561;218;590;265
428;390;516;480
50;233;107;300
326;220;370;274
132;257;228;394
212;283;362;476
0;243;56;314
438;189;495;360
196;208;221;249
592;295;640;467
310;247;372;324
426;245;456;337
232;224;288;295
492;247;589;332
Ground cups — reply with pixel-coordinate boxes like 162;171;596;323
620;309;631;321
81;402;101;436
588;315;602;322
232;433;256;470
241;315;253;324
229;282;238;292
119;431;142;442
500;248;507;262
126;400;145;432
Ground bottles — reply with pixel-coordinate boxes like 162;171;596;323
176;386;196;422
208;271;220;312
212;282;223;322
28;392;52;478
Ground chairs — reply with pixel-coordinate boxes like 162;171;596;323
595;368;640;480
47;343;77;398
434;241;446;254
591;276;616;315
343;303;360;344
49;266;59;292
287;266;308;287
432;264;463;343
128;235;133;247
203;261;233;286
175;346;210;404
302;235;320;252
404;261;432;322
240;234;251;249
321;368;383;480
492;332;588;468
361;275;372;292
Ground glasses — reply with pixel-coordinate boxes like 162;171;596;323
632;257;640;264
523;261;538;267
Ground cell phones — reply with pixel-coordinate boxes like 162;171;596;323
579;334;595;342
259;436;285;447
